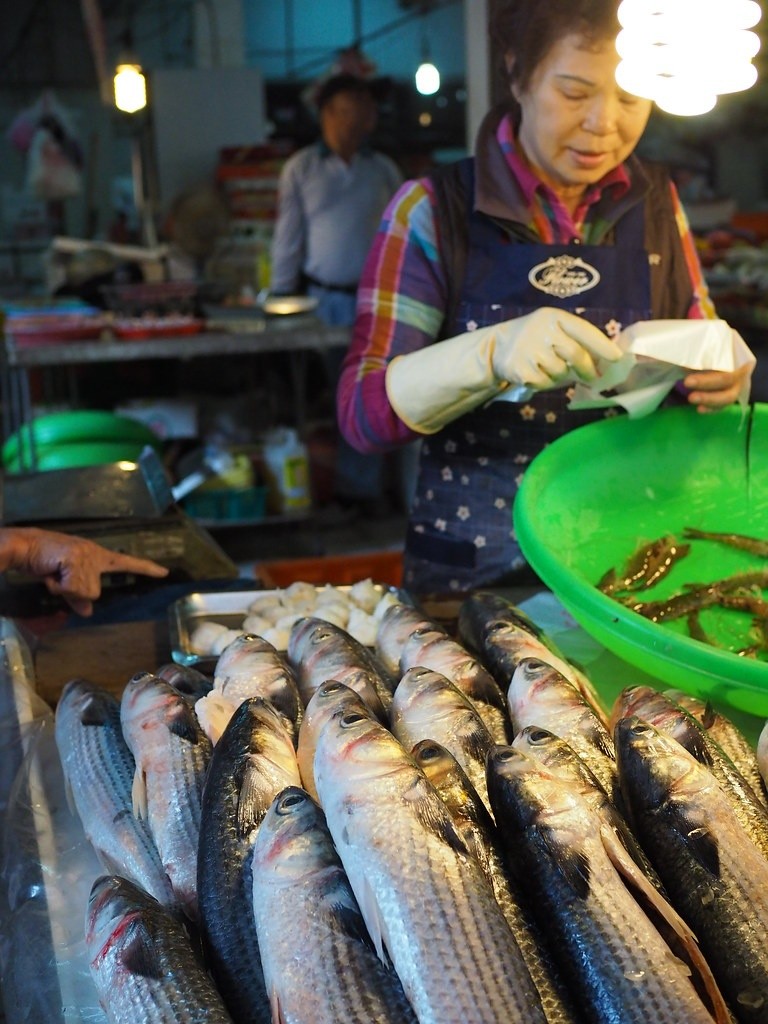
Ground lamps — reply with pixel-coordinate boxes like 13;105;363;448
112;34;147;113
615;0;763;118
414;43;441;96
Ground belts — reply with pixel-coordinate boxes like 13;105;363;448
306;278;356;297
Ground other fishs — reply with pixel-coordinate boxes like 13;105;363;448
52;586;768;1024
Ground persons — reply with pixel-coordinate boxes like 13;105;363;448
0;526;170;619
269;71;420;524
335;1;758;638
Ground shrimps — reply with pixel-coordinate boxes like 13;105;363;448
735;617;768;660
717;583;768;617
683;571;768;595
624;583;725;625
595;535;692;613
683;527;768;559
687;610;711;644
